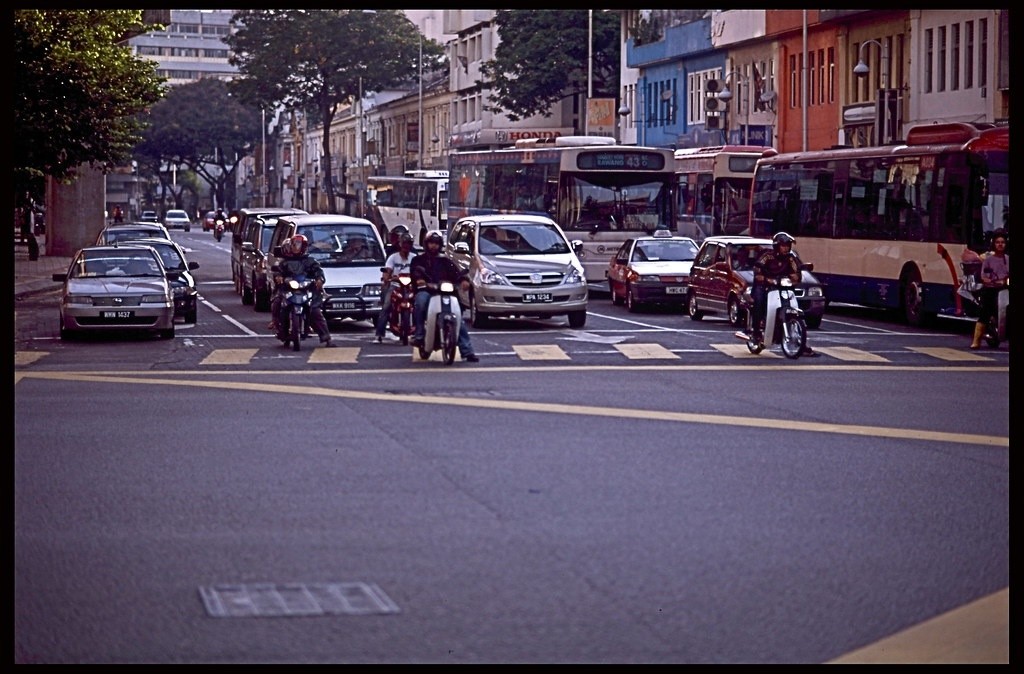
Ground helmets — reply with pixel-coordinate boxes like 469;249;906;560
281;238;292;257
772;231;796;260
390;231;403;251
290;234;308;257
399;232;413;251
425;229;442;257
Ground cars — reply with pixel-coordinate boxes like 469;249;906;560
689;235;826;331
203;212;231;231
606;230;700;313
446;215;589;329
163;210;191;233
51;222;203;339
141;211;159;222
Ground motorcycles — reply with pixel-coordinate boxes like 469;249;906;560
958;249;1011;349
212;220;226;241
734;273;807;360
269;266;318;351
379;269;470;365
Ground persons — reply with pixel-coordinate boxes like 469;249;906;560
970;232;1009;349
703;247;750;276
751;231;802;342
214;207;226;236
410;230;480;362
268;224;418;347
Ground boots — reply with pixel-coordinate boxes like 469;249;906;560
970;322;985;349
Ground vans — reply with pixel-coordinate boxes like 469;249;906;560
266;212;391;331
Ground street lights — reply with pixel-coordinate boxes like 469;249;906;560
719;73;748;145
618;88;645;146
853;39;887;147
431;125;447;166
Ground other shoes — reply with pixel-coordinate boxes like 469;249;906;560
415;332;424;343
279;327;288;339
372;336;382;344
267;321;274;329
326;342;336;347
467;354;479;362
750;330;761;342
801;346;821;357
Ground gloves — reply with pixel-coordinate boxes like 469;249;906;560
314;279;323;291
275;275;284;285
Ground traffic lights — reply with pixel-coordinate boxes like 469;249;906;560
702;78;726;131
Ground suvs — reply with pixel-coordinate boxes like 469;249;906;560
232;208;310;311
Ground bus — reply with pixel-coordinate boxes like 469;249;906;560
448;135;689;286
748;119;1010;327
366;170;448;252
672;145;777;248
